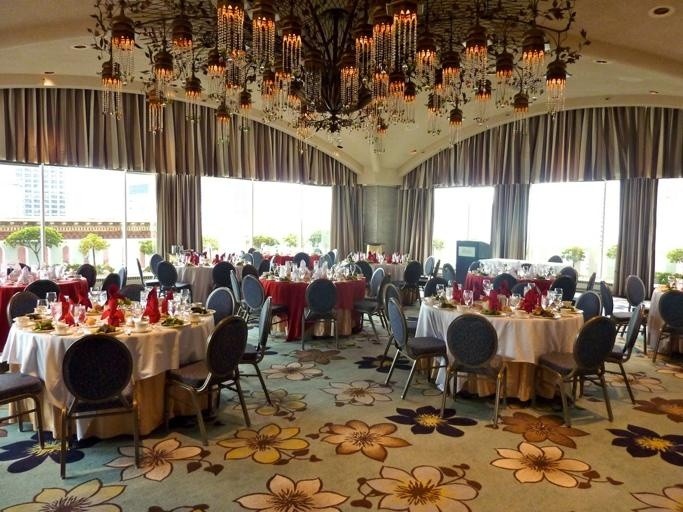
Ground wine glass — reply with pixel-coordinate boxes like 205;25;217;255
351;252;409;263
667;275;676;289
435;279;563;310
169;252;236;265
36;287;190;335
279;260;356;282
481;264;553;277
3;267;70;283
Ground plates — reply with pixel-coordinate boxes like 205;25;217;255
13;307;216;335
422;297;584;317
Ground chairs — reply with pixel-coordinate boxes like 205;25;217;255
652;291;683;362
60;334;140;480
238;296;273;405
1;246;683;357
532;315;617;428
384;298;451;400
440;313;508;428
604;303;646;406
1;373;46;449
163;315;252;446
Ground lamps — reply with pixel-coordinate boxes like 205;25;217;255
79;0;593;156
